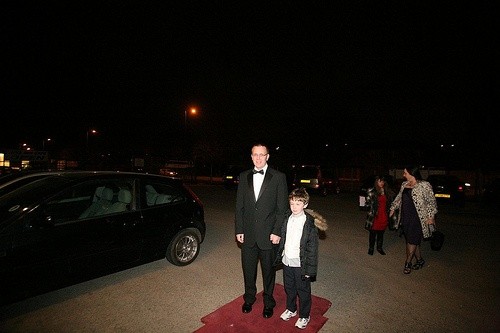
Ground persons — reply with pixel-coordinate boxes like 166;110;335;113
365;175;396;255
389;165;437;274
235;143;289;318
274;186;327;330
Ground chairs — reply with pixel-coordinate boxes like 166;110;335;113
78;186;113;219
104;190;131;214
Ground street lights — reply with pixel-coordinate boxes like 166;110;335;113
86;130;96;155
43;136;51;151
183;106;198;165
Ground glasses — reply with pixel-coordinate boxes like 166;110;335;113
251;153;267;158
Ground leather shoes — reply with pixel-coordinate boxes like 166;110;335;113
263;301;276;318
242;297;256;312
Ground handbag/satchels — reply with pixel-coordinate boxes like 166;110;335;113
427;223;445;252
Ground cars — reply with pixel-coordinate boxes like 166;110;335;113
292;166;320;193
357;174;392;212
0;169;206;307
426;175;466;207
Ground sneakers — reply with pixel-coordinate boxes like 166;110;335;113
294;316;310;329
280;309;297;321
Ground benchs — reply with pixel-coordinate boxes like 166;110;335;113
145;192;176;206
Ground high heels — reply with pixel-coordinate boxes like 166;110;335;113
412;258;425;270
404;260;412;274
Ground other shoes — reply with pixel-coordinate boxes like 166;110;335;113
368;250;374;255
377;250;386;255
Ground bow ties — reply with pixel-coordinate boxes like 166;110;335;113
252;169;264;175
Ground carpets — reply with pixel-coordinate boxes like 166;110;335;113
192;283;331;333
